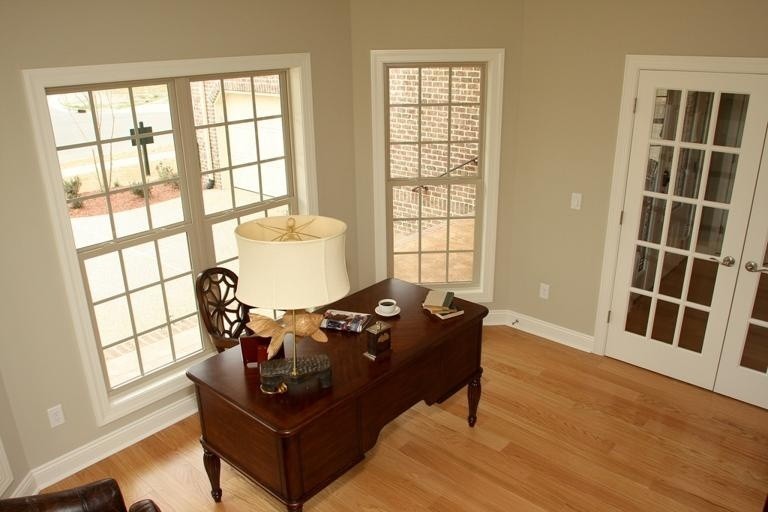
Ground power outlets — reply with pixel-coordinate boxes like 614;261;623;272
539;282;550;300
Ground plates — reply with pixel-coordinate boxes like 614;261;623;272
374;306;401;317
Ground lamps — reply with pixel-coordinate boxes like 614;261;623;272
234;215;351;398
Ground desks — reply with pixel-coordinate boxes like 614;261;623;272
184;276;489;512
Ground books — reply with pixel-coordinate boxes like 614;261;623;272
420;301;464;320
422;290;454;310
319;309;372;333
427;302;456;314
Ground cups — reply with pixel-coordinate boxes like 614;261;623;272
378;298;397;313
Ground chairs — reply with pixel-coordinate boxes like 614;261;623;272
0;478;162;512
194;267;254;354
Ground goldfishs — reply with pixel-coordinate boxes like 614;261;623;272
245;310;328;361
